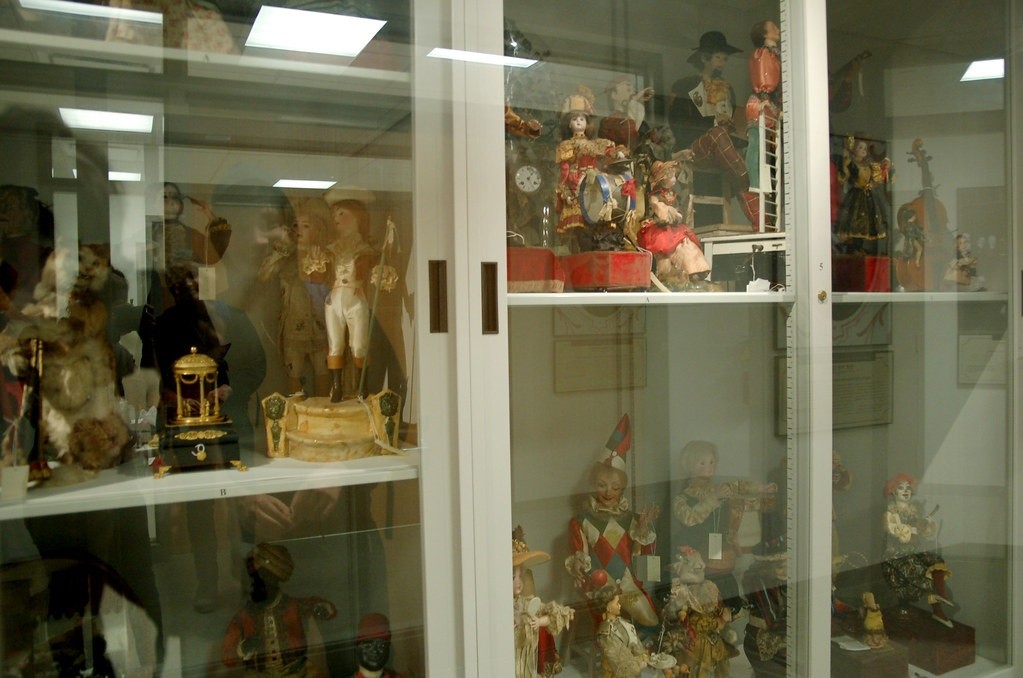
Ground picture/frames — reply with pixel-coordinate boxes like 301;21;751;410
550;184;1008;439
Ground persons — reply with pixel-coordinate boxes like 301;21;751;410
349;613;407;678
222;544;336;678
226;178;401;678
557;20;782;291
835;136;979;278
152;263;264;610
512;415;954;678
30;505;152;678
139;181;397;401
